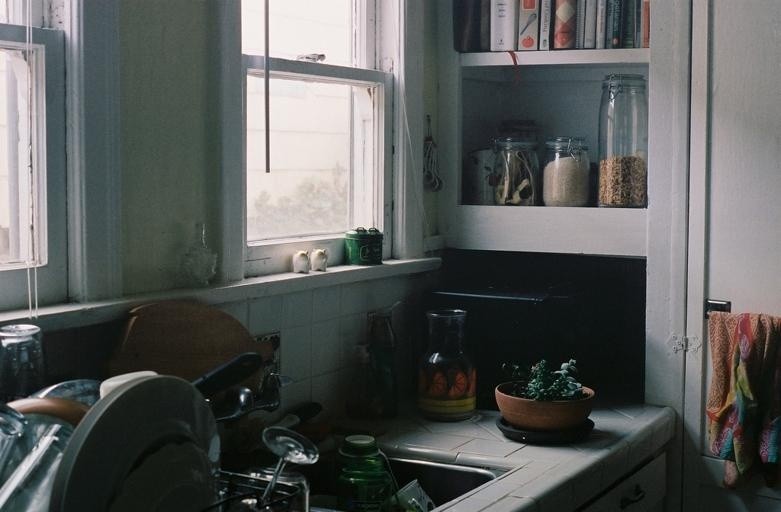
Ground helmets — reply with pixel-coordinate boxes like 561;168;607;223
47;373;222;512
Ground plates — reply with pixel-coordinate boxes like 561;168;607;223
435;0;650;259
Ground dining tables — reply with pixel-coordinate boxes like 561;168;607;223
495;358;596;445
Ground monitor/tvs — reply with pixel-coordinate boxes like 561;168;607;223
455;0;649;52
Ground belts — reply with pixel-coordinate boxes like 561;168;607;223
230;425;321;512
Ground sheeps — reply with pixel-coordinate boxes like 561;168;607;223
217;385;254;428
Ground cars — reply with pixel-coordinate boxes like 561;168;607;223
462;147;496;205
392;478;436;512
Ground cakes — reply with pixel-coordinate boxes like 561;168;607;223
493;137;539;206
541;136;592;207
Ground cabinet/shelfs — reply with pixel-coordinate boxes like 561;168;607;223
492;129;538;205
416;307;478;422
595;72;648;209
346;310;400;419
543;134;589;208
334;434;400;512
